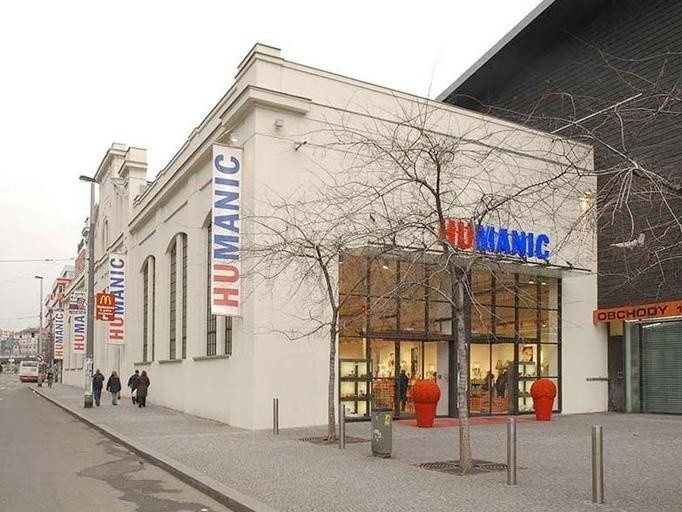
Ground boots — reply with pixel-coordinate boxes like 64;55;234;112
131;396;135;404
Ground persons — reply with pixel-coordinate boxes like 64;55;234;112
387;352;395;367
495;371;505;398
92;368;105;406
523;346;533;362
46;368;54;388
128;369;140;405
106;371;122;405
134;370;151;407
400;369;409;411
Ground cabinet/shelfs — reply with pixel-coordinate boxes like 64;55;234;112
339;358;373;421
509;361;536;416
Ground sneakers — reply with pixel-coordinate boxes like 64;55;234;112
95;401;100;406
138;402;145;407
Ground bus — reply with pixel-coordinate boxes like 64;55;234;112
19;360;39;383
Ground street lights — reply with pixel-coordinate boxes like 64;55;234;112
34;275;44;386
78;175;97;408
